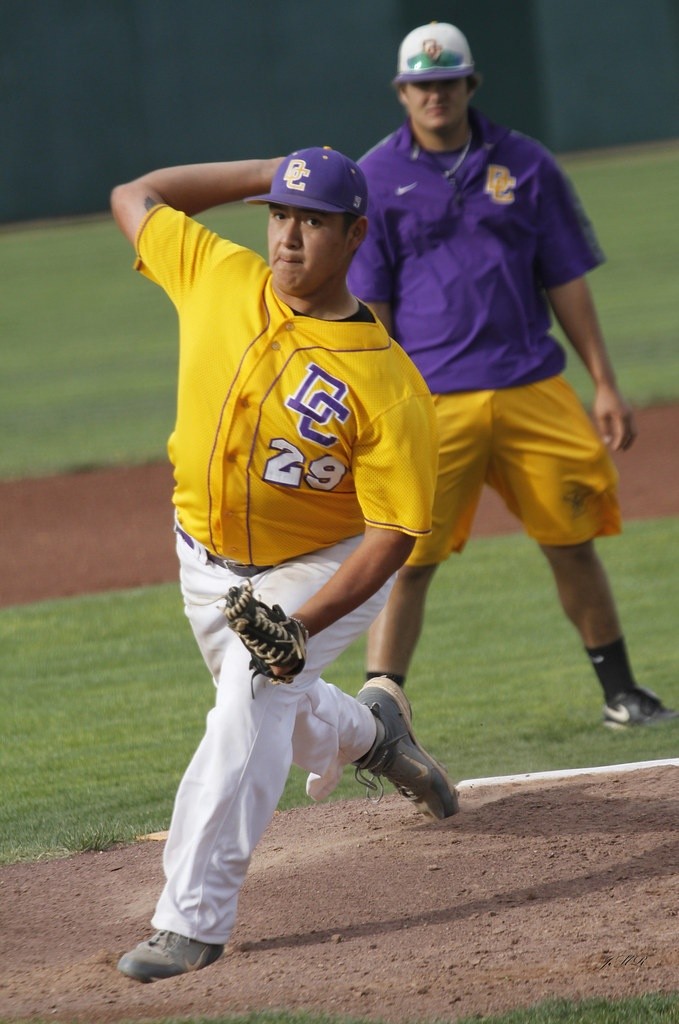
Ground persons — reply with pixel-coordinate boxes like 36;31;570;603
346;19;679;736
111;146;463;987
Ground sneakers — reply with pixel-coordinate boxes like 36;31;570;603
602;686;679;730
116;930;224;983
353;678;459;820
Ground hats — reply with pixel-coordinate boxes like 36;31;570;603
243;145;369;218
391;20;475;82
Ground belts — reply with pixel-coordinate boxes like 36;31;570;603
174;527;273;577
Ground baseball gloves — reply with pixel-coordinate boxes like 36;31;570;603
224;584;306;685
